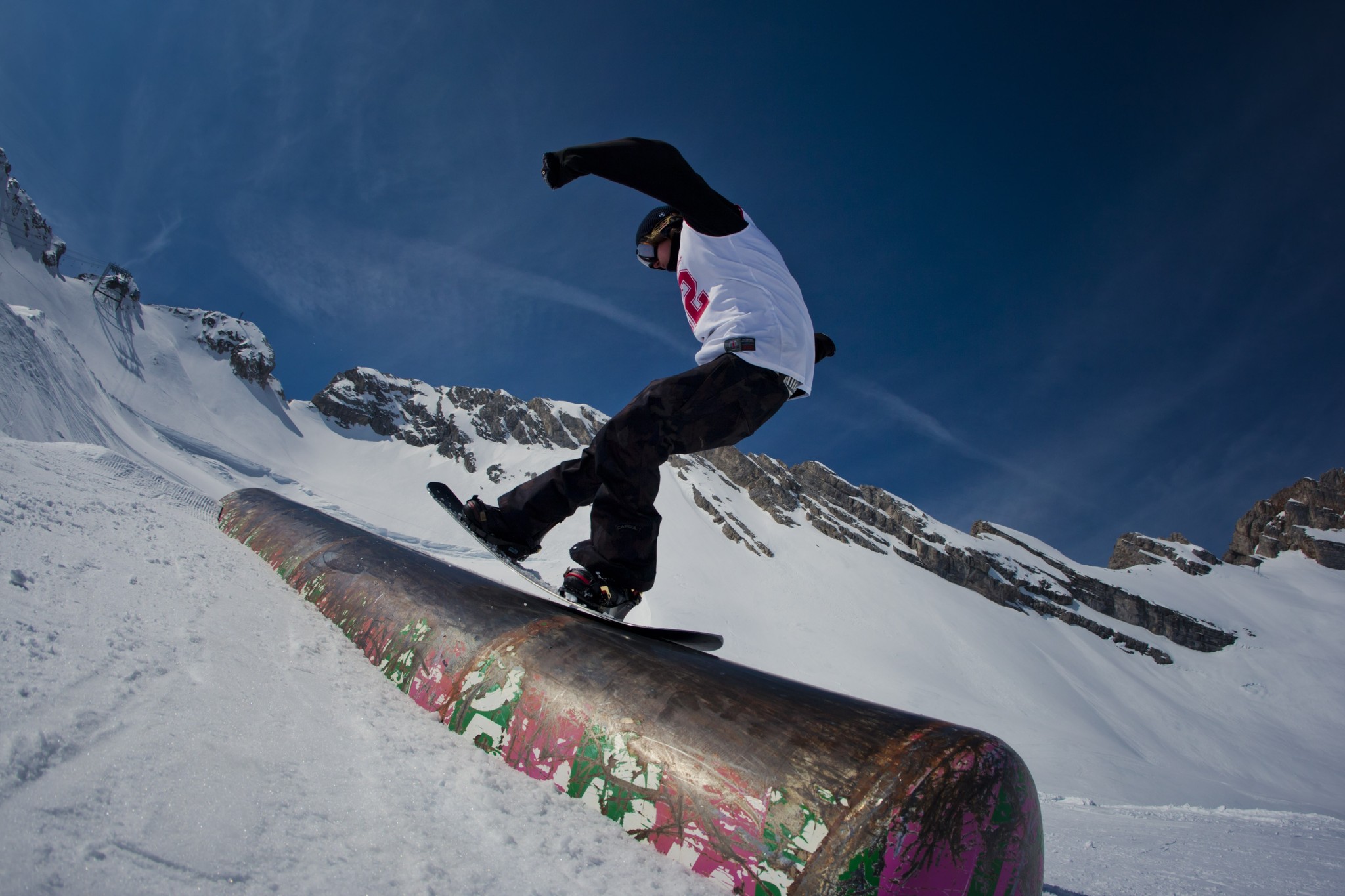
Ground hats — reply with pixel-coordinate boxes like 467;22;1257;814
636;206;681;248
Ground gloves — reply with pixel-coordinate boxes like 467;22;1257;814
541;152;573;191
814;332;836;365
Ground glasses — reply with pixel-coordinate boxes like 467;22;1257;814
636;232;667;271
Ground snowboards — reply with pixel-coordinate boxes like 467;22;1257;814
424;480;725;653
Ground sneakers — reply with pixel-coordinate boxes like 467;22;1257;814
463;494;542;562
563;566;642;608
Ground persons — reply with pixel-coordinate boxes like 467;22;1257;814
463;136;838;622
